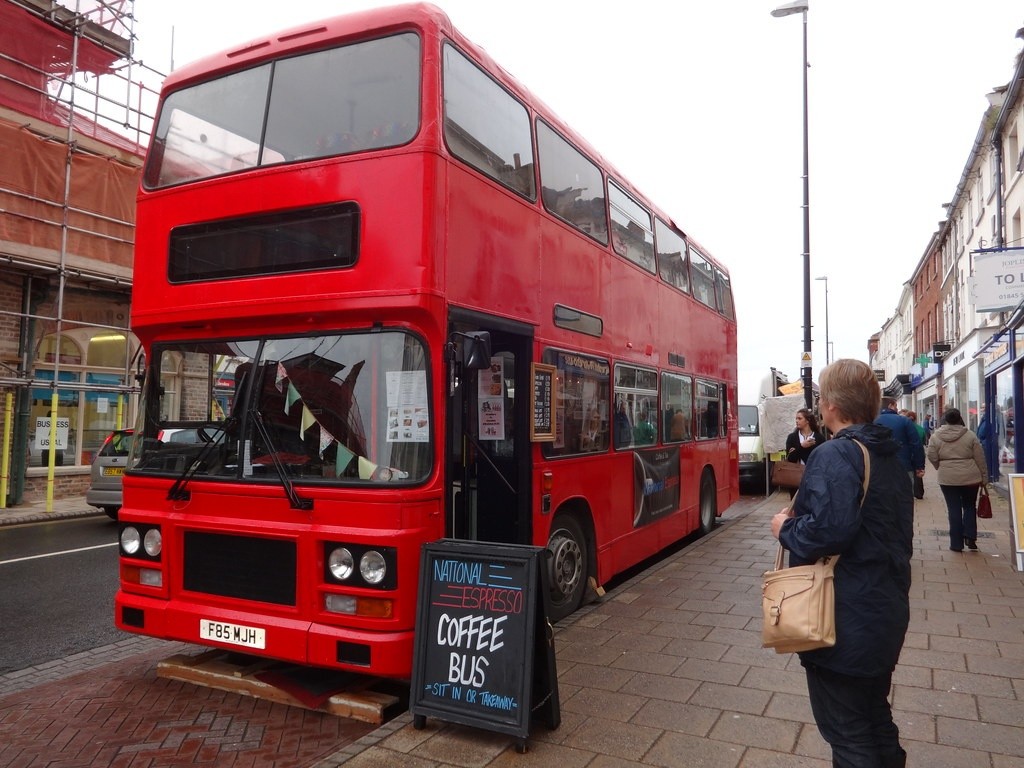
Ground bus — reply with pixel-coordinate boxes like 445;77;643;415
117;0;739;727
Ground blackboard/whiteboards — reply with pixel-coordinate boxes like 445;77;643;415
409;537;559;736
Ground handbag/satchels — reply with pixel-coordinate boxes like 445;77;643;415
770;451;806;488
760;555;836;653
977;485;993;519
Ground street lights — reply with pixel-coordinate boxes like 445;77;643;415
814;277;830;368
827;342;834;364
769;0;814;414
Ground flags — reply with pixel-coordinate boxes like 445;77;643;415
275;361;377;479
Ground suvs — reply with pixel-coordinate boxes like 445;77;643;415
85;428;225;521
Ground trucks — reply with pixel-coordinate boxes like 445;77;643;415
735;357;792;496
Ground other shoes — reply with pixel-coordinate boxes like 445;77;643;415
965;538;977;549
950;546;962;552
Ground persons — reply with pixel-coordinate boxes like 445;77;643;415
633;413;657;444
925;409;988;553
872;398;925;492
579;408;600;447
770;359;914;768
669;409;689;442
784;409;826;501
923;414;933;447
616;401;631;447
976;401;987;448
898;409;926;499
